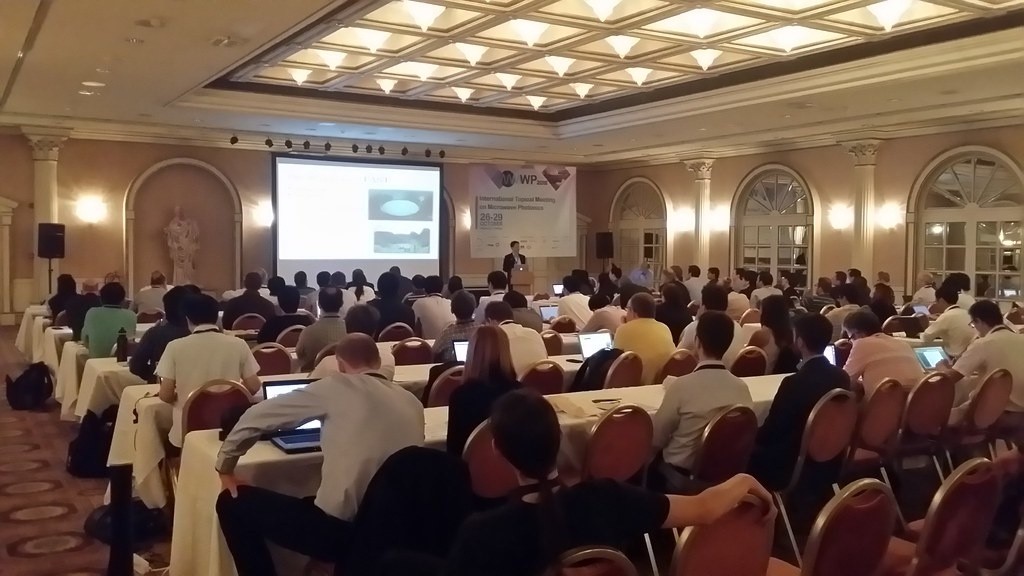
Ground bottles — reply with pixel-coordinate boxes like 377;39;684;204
117;327;128;362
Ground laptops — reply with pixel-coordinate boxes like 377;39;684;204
578;332;613;362
539;305;559;324
553;284;564;295
914;305;931;316
824;344;837;366
514;264;528;272
262;378;324;453
452;339;469;365
912;346;954;374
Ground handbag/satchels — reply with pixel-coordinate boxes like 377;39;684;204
66;410;113;476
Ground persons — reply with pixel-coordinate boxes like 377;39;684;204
49;262;1024;576
503;241;526;290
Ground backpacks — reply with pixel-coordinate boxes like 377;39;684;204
6;361;52;408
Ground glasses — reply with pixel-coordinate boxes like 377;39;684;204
969;316;979;327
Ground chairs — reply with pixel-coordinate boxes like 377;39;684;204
57;304;1023;575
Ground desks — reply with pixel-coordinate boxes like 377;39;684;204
17;294;796;576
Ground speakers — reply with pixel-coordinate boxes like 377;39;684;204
596;232;614;258
38;223;65;258
